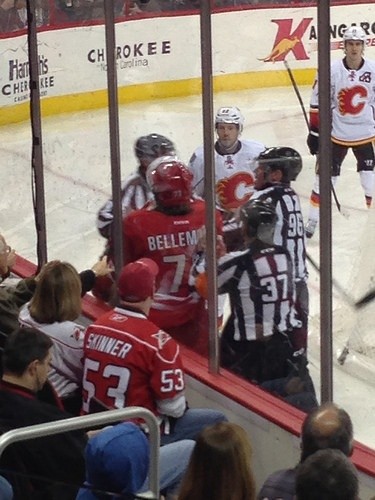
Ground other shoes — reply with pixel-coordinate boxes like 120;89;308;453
303;217;317;239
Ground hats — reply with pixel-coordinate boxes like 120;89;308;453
116;258;159;303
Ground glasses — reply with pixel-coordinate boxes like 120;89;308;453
0;246;11;257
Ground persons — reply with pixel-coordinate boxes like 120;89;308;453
81;257;228;442
1;329;375;500
217;145;310;364
17;260;88;391
0;233;114;337
96;132;176;241
187;106;274;221
305;26;375;239
0;0;172;35
188;198;322;411
104;157;224;355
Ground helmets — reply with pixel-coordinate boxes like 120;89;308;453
240;199;278;246
134;133;175;162
257;147;303;182
214;105;244;133
150;160;194;207
343;26;367;50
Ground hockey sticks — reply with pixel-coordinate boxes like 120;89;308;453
284;59;351;218
305;250;375;309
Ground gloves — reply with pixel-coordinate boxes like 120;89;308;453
306;133;321;156
